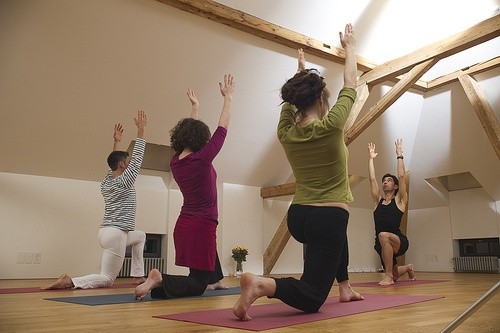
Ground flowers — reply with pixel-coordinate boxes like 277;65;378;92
232;246;248;264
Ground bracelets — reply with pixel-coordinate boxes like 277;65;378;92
397;156;403;159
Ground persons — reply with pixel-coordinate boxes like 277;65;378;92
132;73;235;302
42;109;148;291
367;139;417;286
233;23;365;320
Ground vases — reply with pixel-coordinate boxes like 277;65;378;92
235;263;244;277
